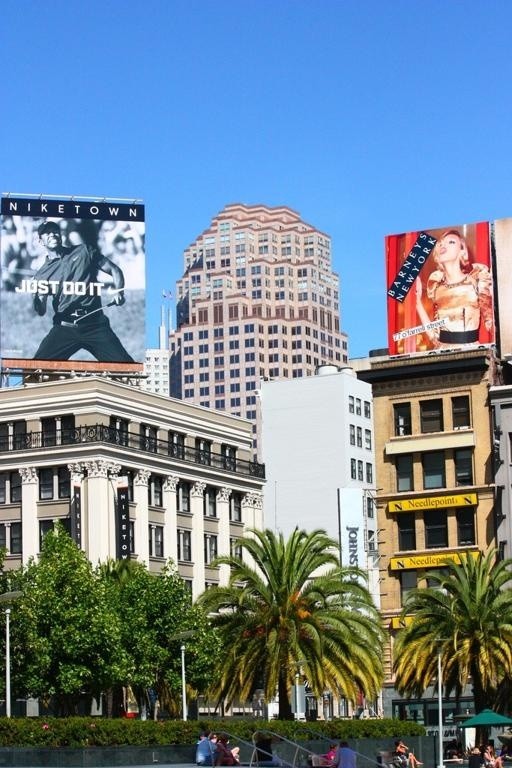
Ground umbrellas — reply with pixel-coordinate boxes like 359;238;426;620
455;707;512;745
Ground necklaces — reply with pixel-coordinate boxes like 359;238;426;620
438;275;469;289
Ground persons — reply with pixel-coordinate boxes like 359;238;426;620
2;213;146;291
196;727;243;767
24;220;141;365
251;731;281;767
394;739;425;768
327;741;358;768
443;730;511;768
324;739;339;768
413;229;494;349
358;706;373;719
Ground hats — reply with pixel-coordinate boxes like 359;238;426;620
38;221;59;238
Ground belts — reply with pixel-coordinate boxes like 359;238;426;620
67;308;98;319
